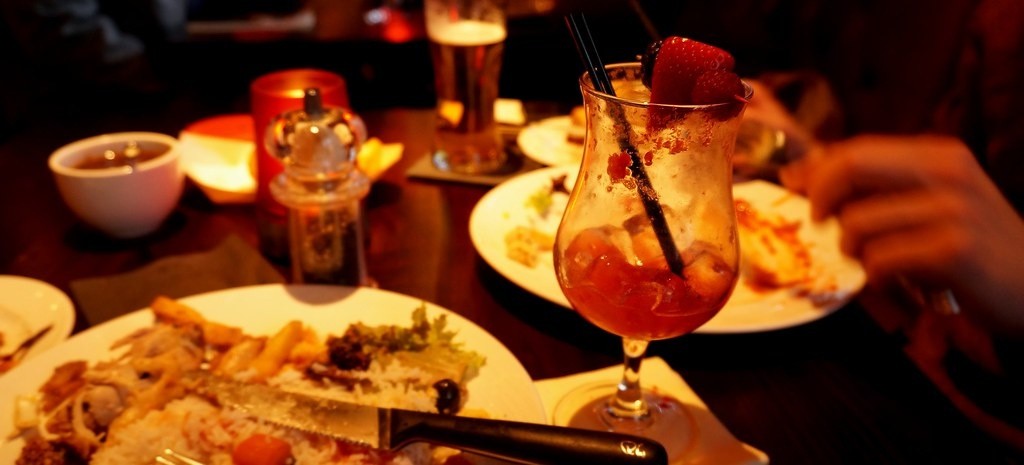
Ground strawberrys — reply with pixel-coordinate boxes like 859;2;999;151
646;35;735;125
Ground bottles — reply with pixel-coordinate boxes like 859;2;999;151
250;70;351;271
270;88;369;285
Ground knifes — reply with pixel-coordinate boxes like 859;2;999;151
182;370;668;465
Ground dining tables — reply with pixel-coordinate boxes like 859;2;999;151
1;100;1024;465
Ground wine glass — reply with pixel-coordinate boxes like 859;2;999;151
554;60;755;465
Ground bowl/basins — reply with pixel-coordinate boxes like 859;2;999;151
48;131;186;239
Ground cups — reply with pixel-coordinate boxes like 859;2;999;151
425;0;508;173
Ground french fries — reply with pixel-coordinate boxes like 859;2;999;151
152;296;330;382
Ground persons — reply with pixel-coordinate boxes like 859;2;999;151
0;0;302;124
776;0;1024;445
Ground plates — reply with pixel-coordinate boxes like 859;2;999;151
468;161;867;334
0;274;76;374
519;117;785;166
0;282;548;465
